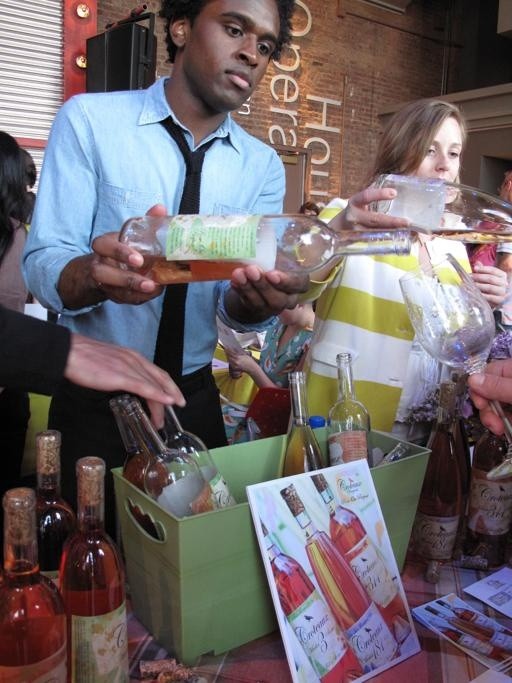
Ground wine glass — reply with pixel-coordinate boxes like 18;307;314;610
399;253;512;480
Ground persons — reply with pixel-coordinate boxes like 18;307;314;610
1;132;190;494
21;1;310;528
226;99;511;434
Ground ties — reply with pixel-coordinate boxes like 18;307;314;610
154;117;216;384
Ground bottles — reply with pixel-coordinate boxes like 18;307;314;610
280;368;323;478
117;214;411;283
0;490;69;681
105;390;241;543
369;172;510;238
62;456;131;683
30;429;74;582
327;351;373;466
